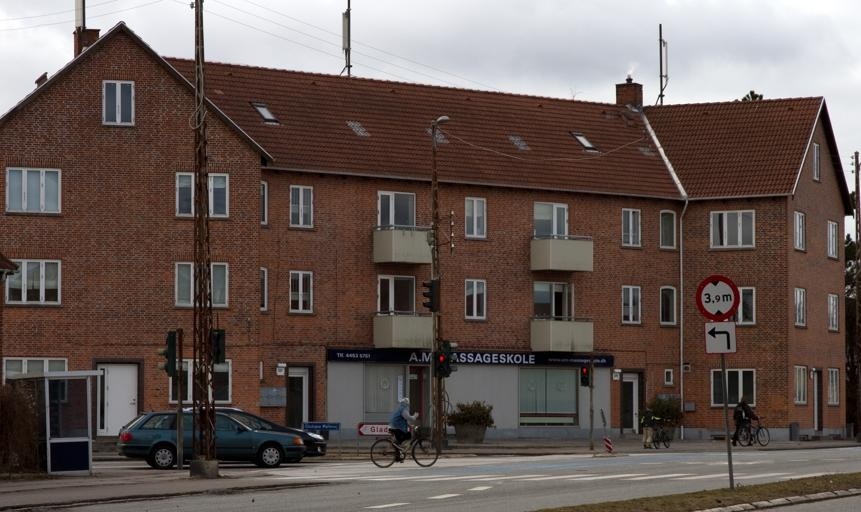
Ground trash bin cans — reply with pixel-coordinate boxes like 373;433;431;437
308;430;329;440
789;423;799;441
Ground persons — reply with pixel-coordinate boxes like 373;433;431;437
732;397;761;447
640;407;663;449
389;397;419;450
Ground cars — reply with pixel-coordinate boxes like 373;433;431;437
116;406;326;469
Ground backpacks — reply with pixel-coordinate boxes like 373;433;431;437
735;406;747;421
643;413;656;427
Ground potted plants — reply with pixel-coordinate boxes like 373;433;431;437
445;400;497;444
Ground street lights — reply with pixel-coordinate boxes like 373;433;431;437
432;115;452;451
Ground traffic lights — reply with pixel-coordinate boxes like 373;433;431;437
580;366;590;386
159;331;176;377
422;279;441;313
435;351;451;379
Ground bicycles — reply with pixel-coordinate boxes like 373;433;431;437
652;423;671;449
737;415;770;446
370;424;440;468
